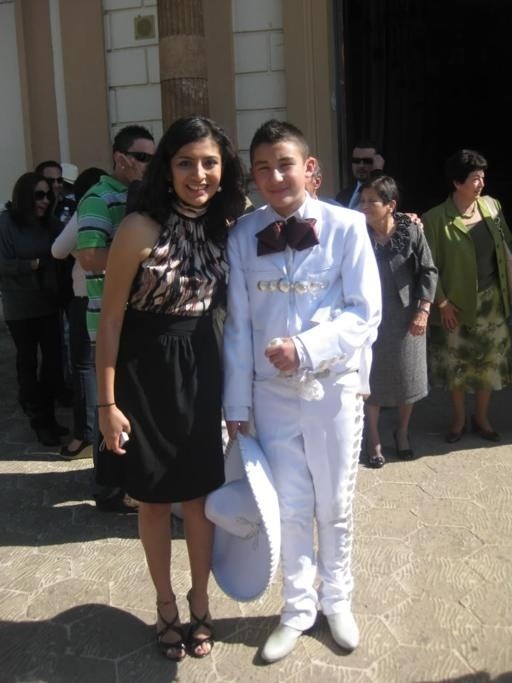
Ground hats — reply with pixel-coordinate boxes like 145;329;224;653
203;428;285;602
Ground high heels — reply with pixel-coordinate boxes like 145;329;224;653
364;438;386;470
61;442;94;461
390;429;417;459
444;415;503;442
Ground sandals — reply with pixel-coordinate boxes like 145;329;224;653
156;598;214;659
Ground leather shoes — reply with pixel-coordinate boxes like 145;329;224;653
322;601;361;654
259;621;304;662
31;419;71;447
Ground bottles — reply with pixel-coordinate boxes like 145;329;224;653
62;206;71;224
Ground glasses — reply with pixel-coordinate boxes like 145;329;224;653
352;154;373;165
32;190;56;199
46;175;64;184
124;149;154;162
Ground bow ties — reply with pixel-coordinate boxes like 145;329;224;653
252;216;323;256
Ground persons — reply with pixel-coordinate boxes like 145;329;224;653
220;120;384;662
95;114;248;662
1;125;510;509
